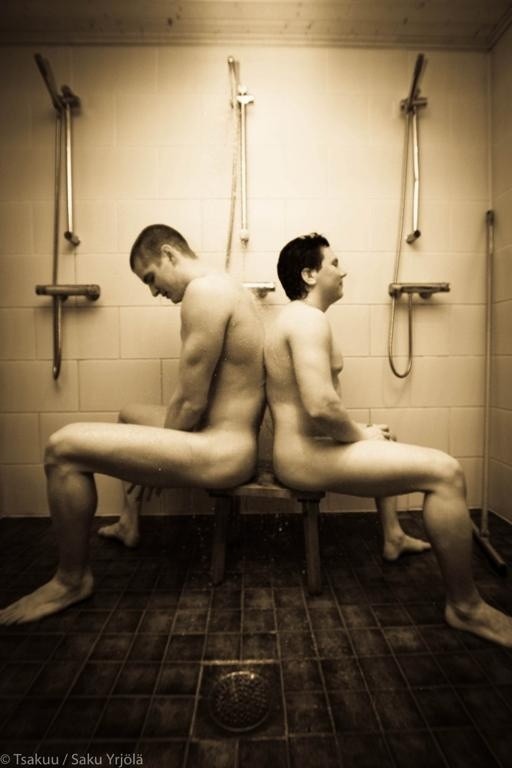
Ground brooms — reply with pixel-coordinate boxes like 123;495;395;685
469;208;509;573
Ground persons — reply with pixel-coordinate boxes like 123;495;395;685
261;231;511;650
0;221;266;626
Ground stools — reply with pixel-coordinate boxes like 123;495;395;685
205;459;328;597
